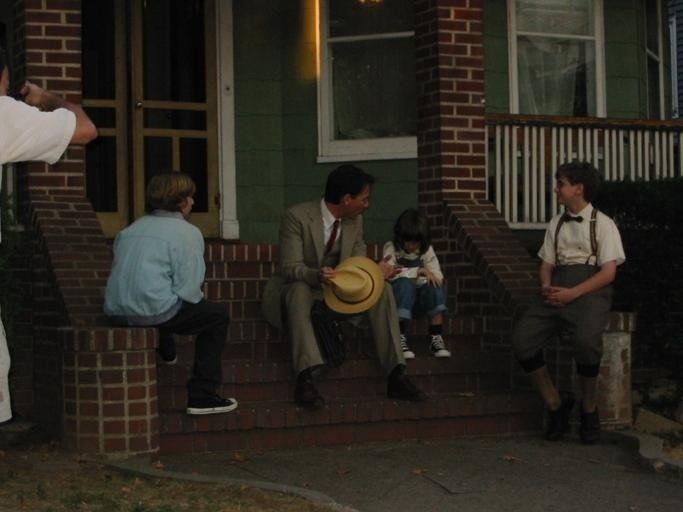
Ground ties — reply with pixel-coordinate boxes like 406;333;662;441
326;220;339;253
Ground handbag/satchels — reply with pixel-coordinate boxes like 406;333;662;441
311;304;344;369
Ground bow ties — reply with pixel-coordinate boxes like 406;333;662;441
563;215;584;222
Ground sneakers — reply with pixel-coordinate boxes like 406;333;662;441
400;335;415;358
429;335;451;357
187;396;238;414
158;339;178;366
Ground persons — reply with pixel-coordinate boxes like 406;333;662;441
512;162;626;444
102;166;238;415
1;54;99;422
261;163;427;408
383;207;450;359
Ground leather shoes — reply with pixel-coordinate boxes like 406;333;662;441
388;374;426;402
581;407;600;442
546;391;574;441
296;383;324;408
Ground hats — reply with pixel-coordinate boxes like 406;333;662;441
322;255;384;313
